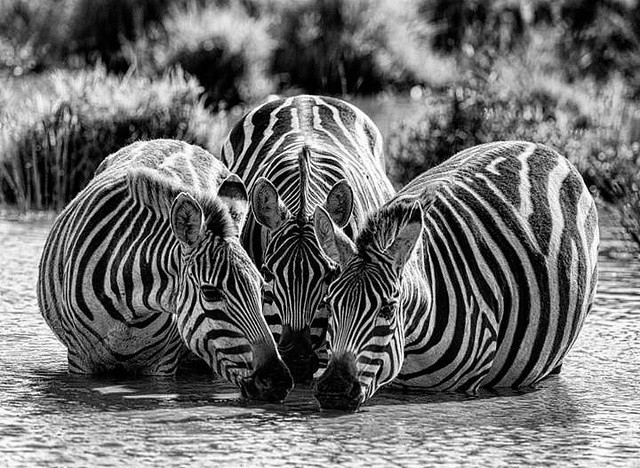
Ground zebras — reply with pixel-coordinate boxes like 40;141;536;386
37;139;293;402
220;95;397;373
314;140;598;413
